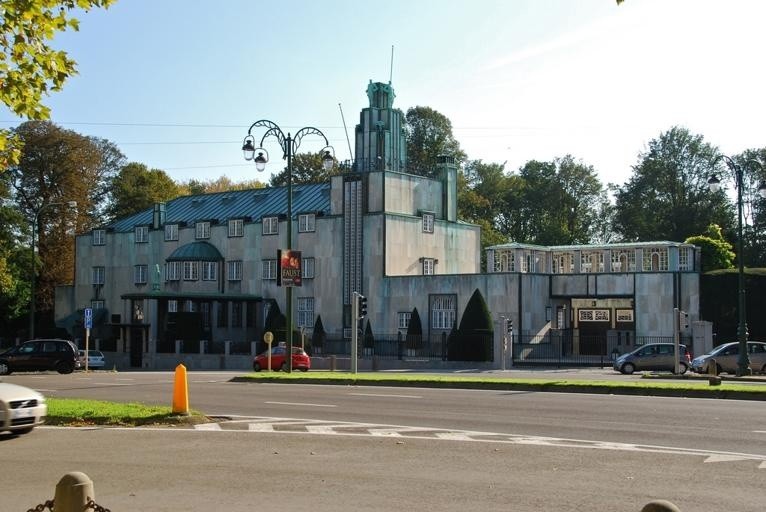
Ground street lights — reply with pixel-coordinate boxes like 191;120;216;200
242;119;334;375
29;199;81;340
708;152;765;377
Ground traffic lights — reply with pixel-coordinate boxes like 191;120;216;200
355;295;367;321
505;319;512;333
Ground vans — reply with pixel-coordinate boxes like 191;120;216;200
691;340;765;378
1;338;80;375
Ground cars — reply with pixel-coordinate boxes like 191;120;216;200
613;343;690;375
78;349;105;370
253;342;311;372
0;382;47;439
